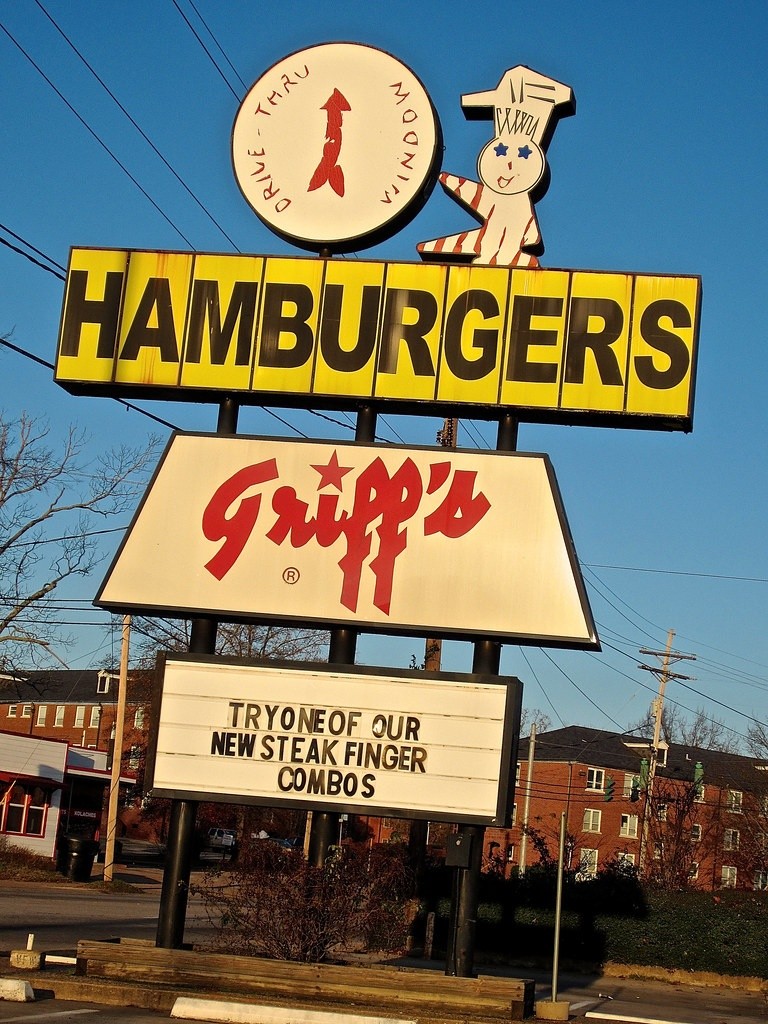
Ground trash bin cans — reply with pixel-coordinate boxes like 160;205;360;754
61;834;101;883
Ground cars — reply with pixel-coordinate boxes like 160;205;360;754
270;837;304;846
207;828;238;846
